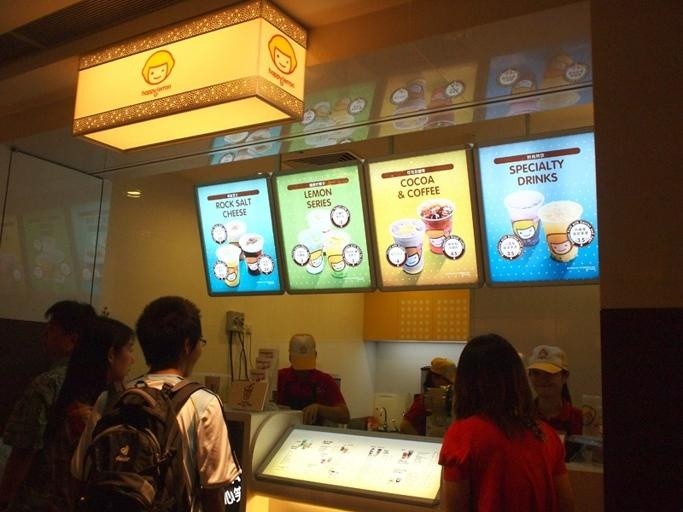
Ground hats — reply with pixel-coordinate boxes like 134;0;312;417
430;356;458;385
525;344;570;376
287;333;319;371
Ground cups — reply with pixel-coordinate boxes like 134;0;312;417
216;54;583;288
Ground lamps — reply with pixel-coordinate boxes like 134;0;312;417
71;1;309;155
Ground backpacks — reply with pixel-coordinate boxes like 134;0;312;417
83;380;208;512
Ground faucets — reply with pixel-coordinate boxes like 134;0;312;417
379;407;387;423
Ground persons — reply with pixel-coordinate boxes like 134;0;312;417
70;294;241;510
527;345;584;462
399;357;457;436
437;332;579;511
0;300;95;509
270;332;350;427
16;316;135;511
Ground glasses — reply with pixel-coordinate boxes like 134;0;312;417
196;333;206;347
